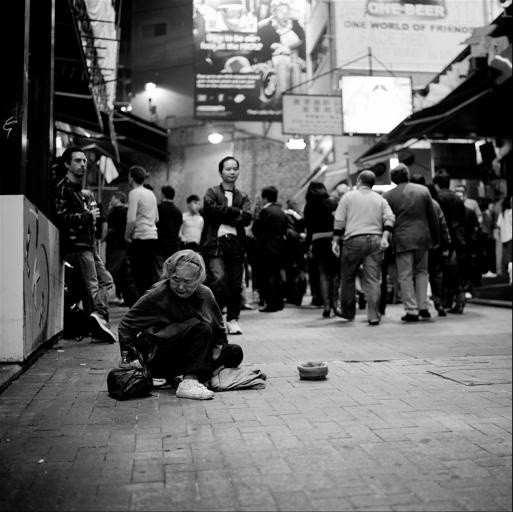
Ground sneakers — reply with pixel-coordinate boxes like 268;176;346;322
89;309;115;342
400;315;418;321
175;380;215;399
226;321;240;335
420;312;431;318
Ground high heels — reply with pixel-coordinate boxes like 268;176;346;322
321;309;330;318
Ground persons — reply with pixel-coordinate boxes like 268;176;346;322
331;170;396;326
100;191;128;306
124;165;159;294
250;186;288;313
155;185;183;251
431;173;467;314
454;185;512;286
117;250;243;399
408;172;451;316
380;163;440;322
179;195;204;253
282;198;305;306
256;0;305;96
300;180;341;318
54;146;116;344
198;156;252;335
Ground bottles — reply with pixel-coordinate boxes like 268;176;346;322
90;192;96;209
